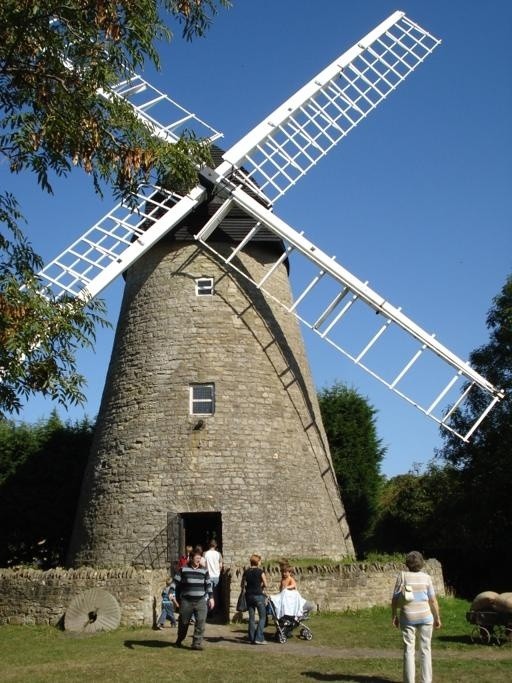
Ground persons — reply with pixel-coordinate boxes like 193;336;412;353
240;554;270;645
279;564;297;637
155;537;223;630
167;547;216;650
391;549;442;683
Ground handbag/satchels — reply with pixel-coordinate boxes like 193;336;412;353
237;591;248;611
396;585;415;608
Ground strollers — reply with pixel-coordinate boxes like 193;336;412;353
261;588;317;644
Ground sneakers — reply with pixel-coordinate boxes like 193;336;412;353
255;640;268;644
